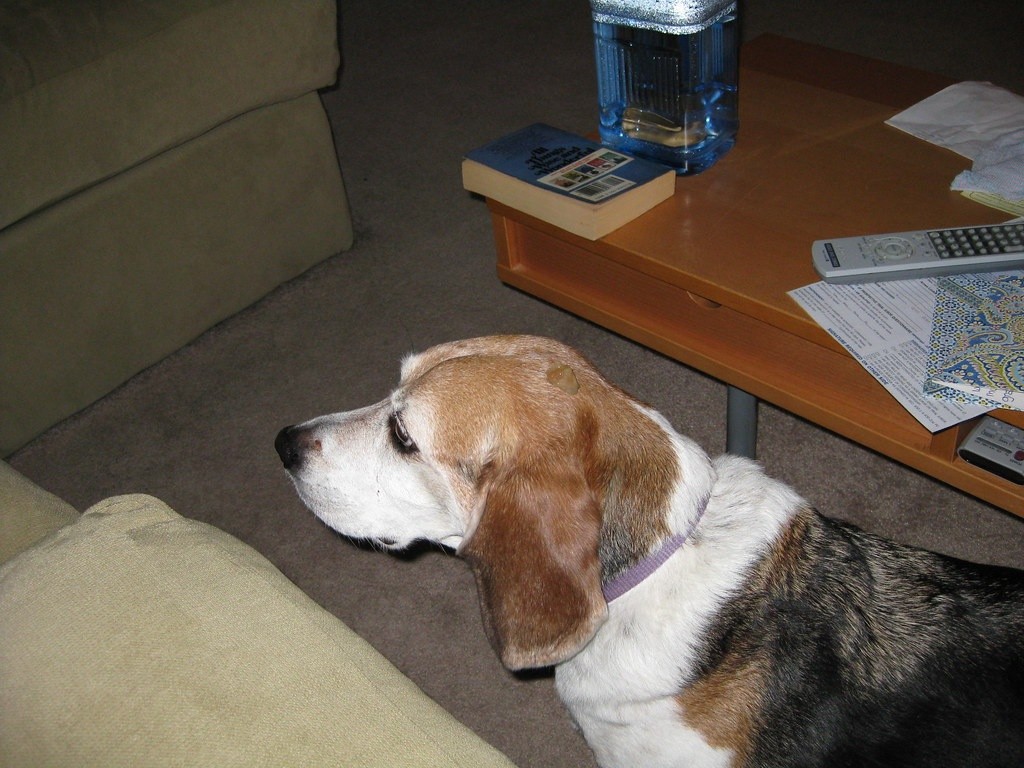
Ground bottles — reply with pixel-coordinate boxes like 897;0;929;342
590;0;739;176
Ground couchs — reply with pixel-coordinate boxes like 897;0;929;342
0;0;352;462
0;451;522;768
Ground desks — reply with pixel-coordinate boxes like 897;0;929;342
486;30;1024;522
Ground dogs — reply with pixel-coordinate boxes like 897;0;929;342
273;331;1024;768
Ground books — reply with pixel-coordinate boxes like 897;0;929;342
461;122;676;241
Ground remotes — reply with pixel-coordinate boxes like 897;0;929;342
957;416;1024;486
812;220;1024;284
